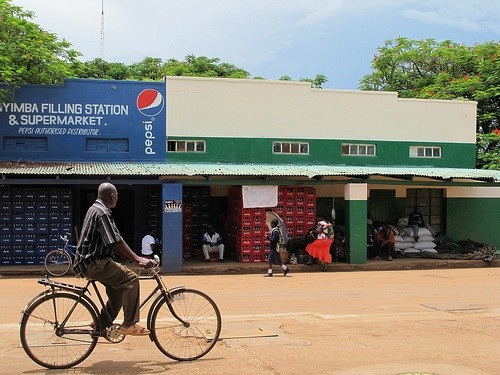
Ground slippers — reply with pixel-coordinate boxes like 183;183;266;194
116;322;150;336
90;322;96;329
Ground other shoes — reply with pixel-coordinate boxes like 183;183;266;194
415;237;419;242
219;259;223;262
435;233;440;237
204;259;210;263
304;260;313;264
388;256;392;261
316;261;324;265
375;256;382;260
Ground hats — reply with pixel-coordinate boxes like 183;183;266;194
207;225;212;229
273;219;278;225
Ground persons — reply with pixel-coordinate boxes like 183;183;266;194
263;218;289;277
375;221;395;261
202;225;225;263
73;183;154;335
407;207;425;242
304;214;334;272
142;228;161;266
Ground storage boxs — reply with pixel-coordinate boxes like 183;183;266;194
0;184;74;265
143;187;221;262
225;185;317;262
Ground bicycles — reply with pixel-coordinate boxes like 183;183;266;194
19;256;222;369
44;231;81;277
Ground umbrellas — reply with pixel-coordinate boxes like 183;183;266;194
266;212;289;248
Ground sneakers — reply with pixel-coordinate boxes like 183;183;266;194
284;267;291;276
263;273;273;277
